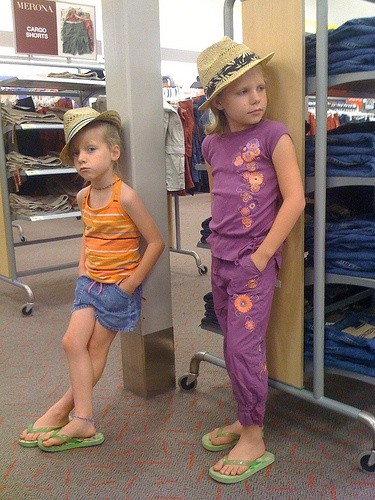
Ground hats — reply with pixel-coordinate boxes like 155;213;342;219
58;107;121;161
197;37;275;110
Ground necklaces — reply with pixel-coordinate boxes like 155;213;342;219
91;176;120;190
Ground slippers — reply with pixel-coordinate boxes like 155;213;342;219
38;429;104;452
209;451;274;483
202;426;264;452
19;416;72;448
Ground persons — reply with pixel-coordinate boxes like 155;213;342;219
18;108;165;453
196;38;305;483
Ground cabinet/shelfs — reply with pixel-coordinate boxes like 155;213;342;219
0;76;208;316
179;0;375;476
